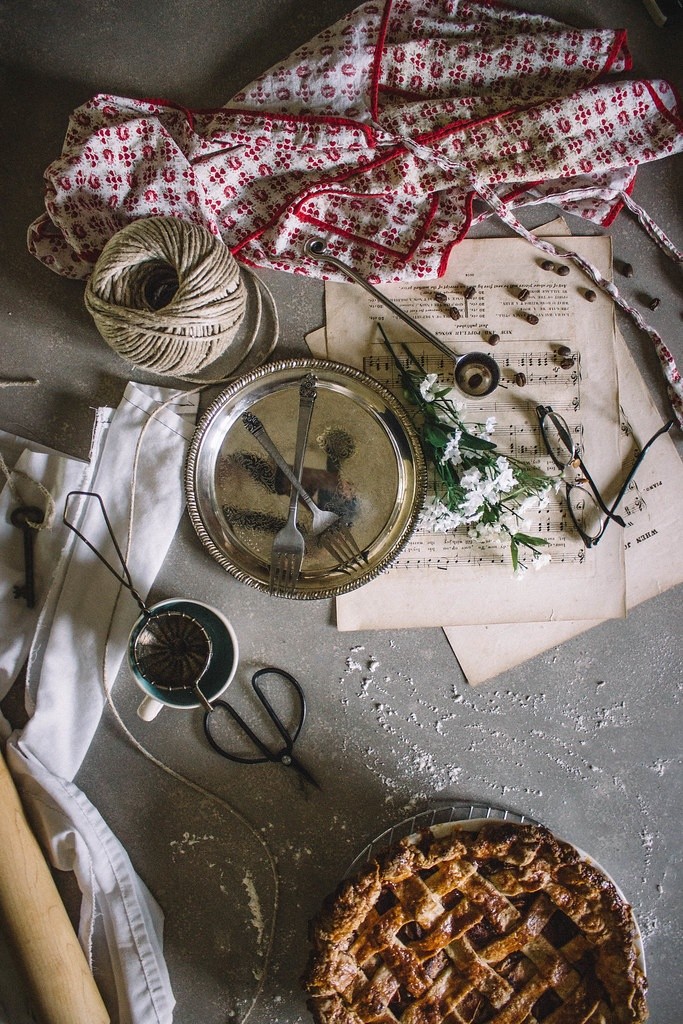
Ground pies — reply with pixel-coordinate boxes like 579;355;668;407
303;825;651;1024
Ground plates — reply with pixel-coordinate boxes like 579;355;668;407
399;818;647;996
183;356;428;601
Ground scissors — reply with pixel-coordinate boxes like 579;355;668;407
203;667;323;801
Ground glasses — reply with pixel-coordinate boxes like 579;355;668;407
535;405;675;548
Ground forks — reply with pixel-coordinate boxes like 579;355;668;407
267;372;317;599
242;410;365;568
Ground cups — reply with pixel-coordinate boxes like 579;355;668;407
126;596;239;722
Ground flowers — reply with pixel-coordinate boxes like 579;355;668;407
365;325;553;579
